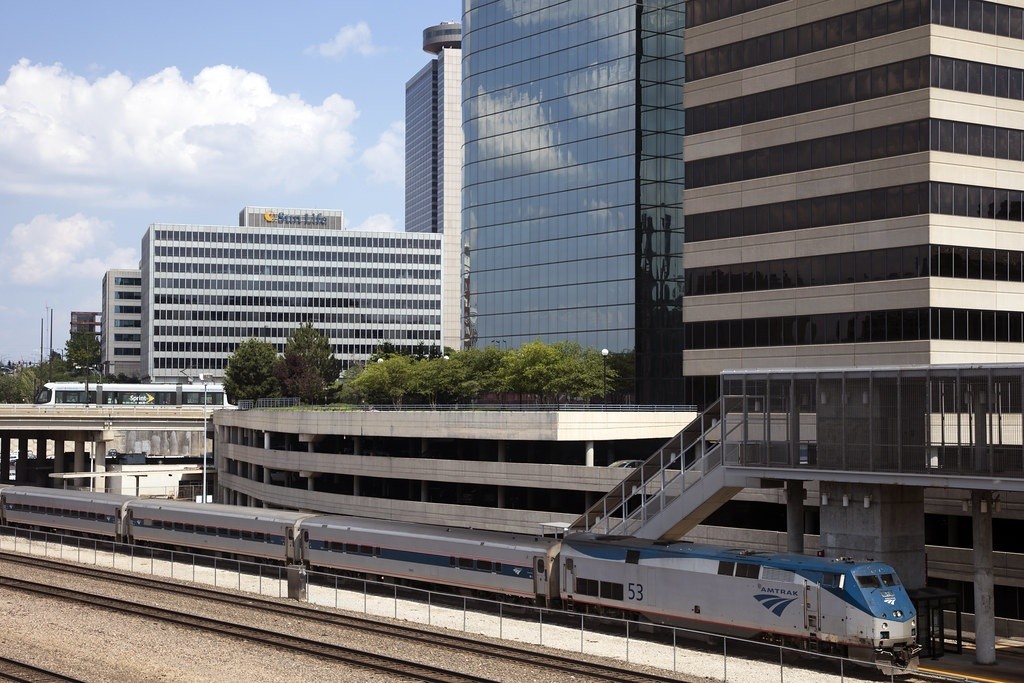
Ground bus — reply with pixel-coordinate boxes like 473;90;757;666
33;382;229;405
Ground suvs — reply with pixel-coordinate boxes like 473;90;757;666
9;448;34;464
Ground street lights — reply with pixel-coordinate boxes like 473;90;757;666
601;349;609;403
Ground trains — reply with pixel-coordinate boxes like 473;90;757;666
0;483;923;675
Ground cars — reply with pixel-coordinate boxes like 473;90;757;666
108;449;117;456
